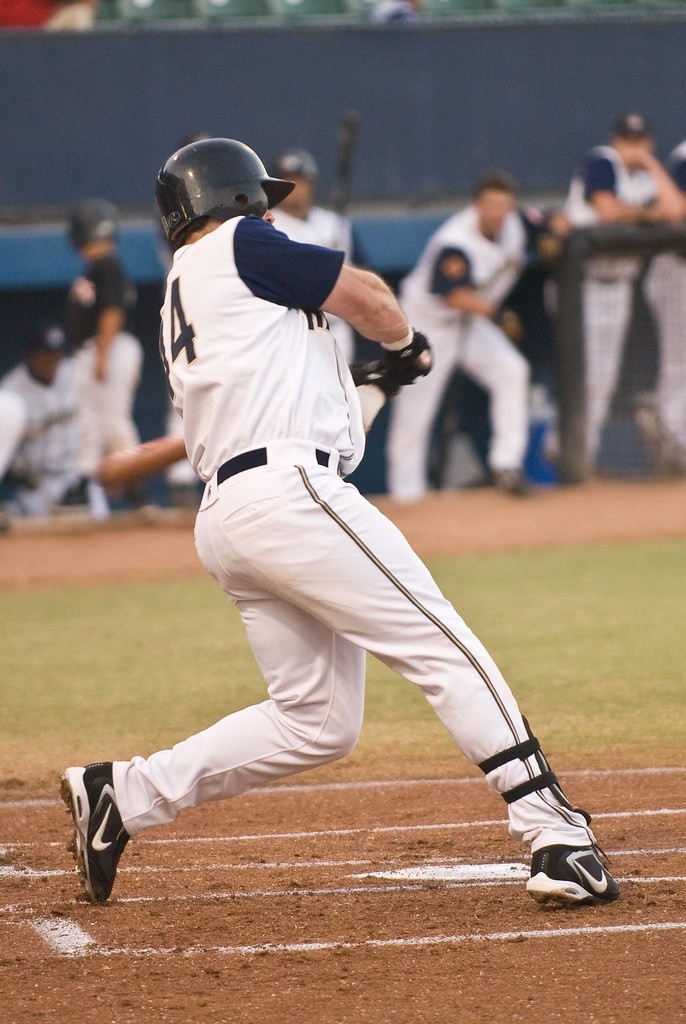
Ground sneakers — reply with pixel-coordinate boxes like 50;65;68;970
60;761;131;906
525;844;621;903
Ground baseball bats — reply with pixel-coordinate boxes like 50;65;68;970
89;344;437;498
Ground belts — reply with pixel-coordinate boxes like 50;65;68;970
216;446;329;485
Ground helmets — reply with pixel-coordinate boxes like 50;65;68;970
154;138;295;252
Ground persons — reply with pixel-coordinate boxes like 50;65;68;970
61;138;621;908
1;113;685;533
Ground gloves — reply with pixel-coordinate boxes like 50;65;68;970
350;328;435;396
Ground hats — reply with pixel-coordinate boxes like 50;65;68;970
26;317;74;351
271;147;320;181
614;109;655;135
474;169;516;191
